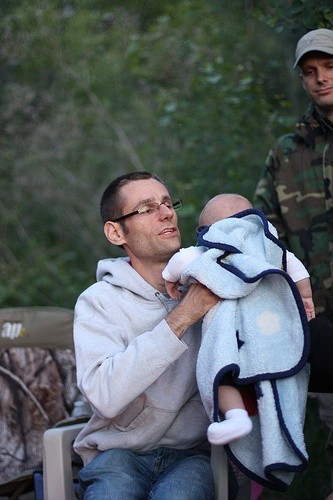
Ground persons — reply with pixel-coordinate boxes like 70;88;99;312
251;29;333;393
162;193;316;444
72;172;223;500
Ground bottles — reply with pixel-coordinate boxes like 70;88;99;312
69;401;90;464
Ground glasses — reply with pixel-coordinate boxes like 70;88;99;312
113;195;183;222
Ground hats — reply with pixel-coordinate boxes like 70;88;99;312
292;28;333;70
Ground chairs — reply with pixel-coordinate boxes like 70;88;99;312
41;420;230;500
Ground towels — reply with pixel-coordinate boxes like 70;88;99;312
177;208;310;492
